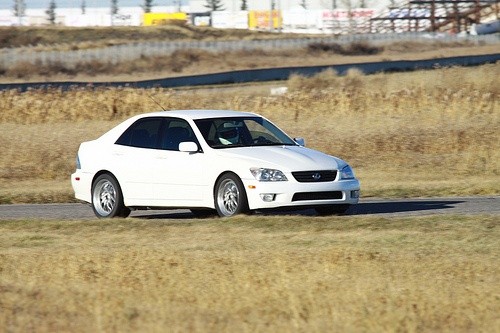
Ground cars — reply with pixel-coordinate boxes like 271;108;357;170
70;111;360;218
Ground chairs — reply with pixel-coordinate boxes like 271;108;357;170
158;126;189;149
